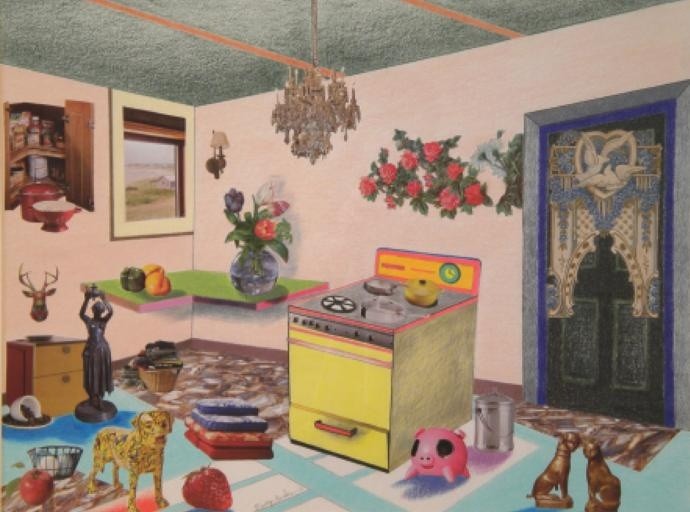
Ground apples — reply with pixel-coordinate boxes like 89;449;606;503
20;468;53;505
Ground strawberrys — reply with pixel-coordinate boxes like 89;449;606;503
182;463;233;511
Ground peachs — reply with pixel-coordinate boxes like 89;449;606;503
141;265;171;296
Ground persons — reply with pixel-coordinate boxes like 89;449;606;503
79;283;115;412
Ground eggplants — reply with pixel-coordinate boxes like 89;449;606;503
128;341;183;370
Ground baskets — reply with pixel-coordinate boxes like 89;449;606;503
29;446;83;477
